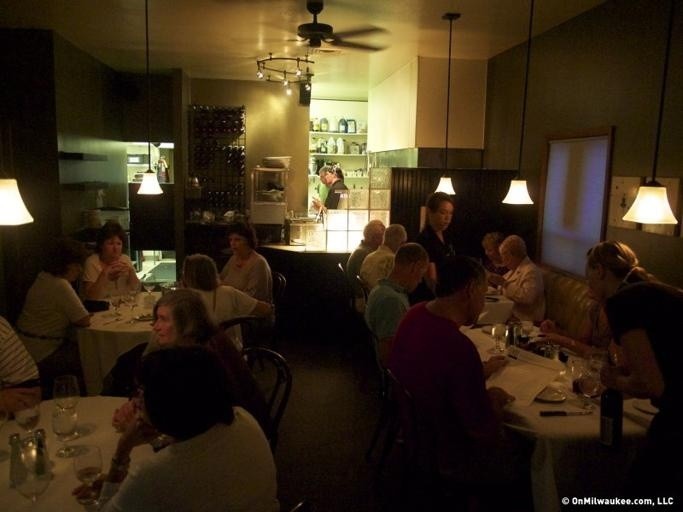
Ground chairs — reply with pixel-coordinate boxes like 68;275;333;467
272;271;287;301
357;276;368;304
338;264;357;368
359;313;395;461
217;315;266;374
239;345;293;442
384;369;468;511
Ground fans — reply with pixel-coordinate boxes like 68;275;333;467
235;0;389;54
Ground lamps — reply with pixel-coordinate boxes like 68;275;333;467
502;1;535;206
622;0;680;228
136;1;164;195
0;177;34;227
256;52;314;95
433;12;461;195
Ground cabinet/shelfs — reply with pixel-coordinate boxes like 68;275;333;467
309;128;368;190
190;106;245;211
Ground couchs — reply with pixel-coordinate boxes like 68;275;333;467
525;261;604;343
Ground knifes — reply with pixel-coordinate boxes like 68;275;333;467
538;411;592;417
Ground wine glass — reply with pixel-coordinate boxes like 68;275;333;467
51;401;78;459
128;295;138;327
74;444;103;510
490;316;506;353
53;374;81;441
11;433;52;511
111;289;121;316
568;358;602;408
142;276;156;295
14;395;41;433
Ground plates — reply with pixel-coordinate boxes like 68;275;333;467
133;312;152;321
535;384;566;402
632;398;660;414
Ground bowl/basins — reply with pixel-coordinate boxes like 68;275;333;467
262;156;292;168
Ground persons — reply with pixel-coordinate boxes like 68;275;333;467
311;167;348;210
1;192;683;511
314;166;329;204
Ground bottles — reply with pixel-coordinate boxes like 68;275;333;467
8;433;26;488
34;429;49;474
312;117;321;131
